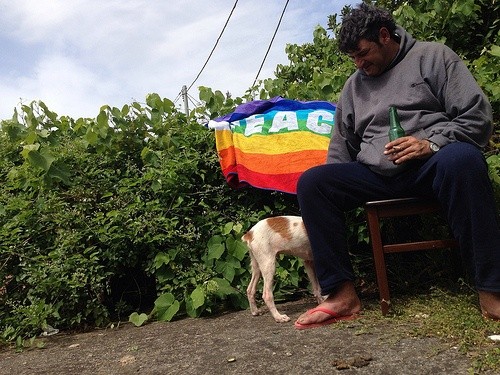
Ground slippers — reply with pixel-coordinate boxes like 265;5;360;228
295;305;355;329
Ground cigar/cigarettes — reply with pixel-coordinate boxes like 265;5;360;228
393;146;401;149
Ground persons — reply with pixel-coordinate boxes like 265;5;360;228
295;3;500;330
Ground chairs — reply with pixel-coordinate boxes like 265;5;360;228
365;196;458;316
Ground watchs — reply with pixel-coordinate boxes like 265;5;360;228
430;143;440;152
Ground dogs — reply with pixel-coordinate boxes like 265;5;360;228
241;215;327;323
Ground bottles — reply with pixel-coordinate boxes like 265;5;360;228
389;107;406;165
